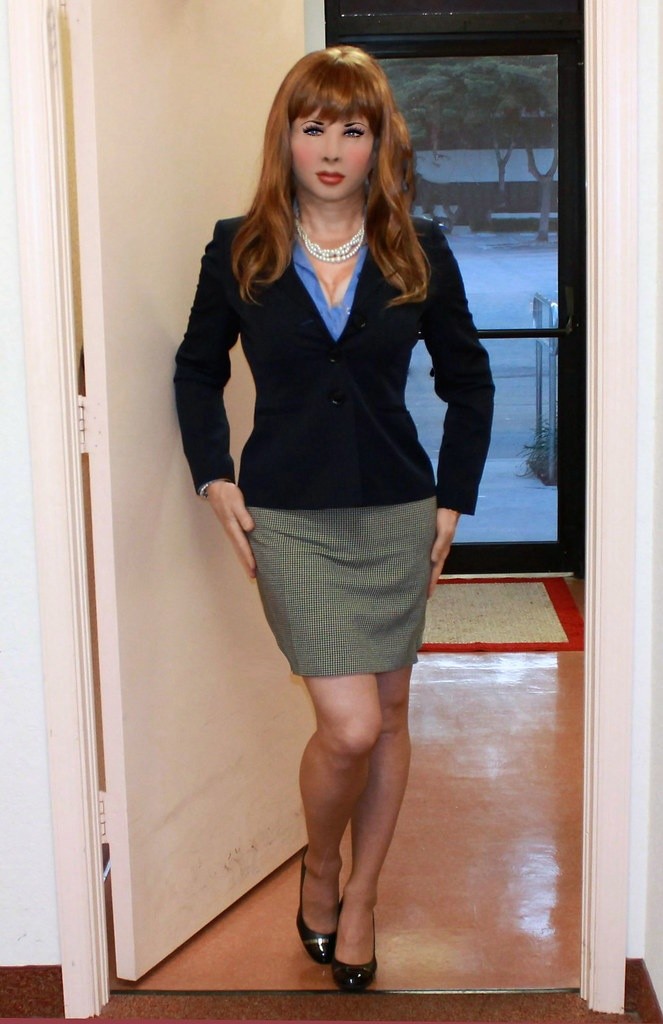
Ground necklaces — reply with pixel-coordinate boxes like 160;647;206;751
292;192;366;262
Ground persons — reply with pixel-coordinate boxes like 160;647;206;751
172;47;496;992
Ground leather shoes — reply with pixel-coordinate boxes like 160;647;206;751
331;893;377;993
296;847;341;964
